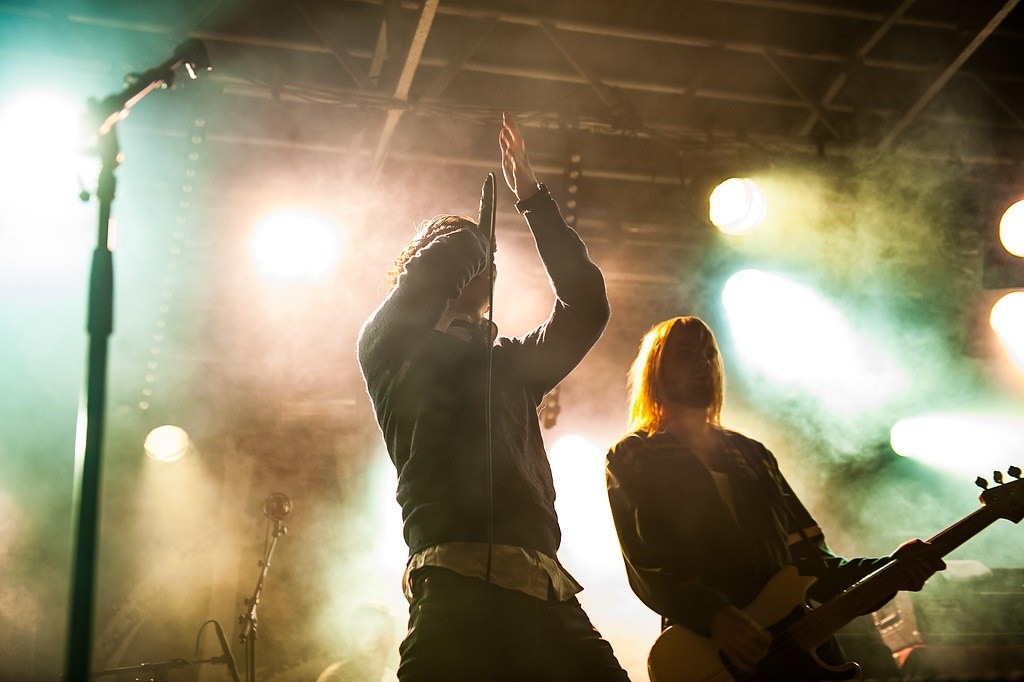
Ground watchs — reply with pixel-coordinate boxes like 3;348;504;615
516;182;553;213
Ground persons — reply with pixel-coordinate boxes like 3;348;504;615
357;111;631;682
607;317;947;667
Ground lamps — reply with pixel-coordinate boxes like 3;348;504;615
682;164;770;243
139;403;189;462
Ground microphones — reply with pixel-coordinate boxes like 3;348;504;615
266;496;289;515
478;176;494;261
214;620;240;682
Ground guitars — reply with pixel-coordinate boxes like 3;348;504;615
646;461;1024;681
